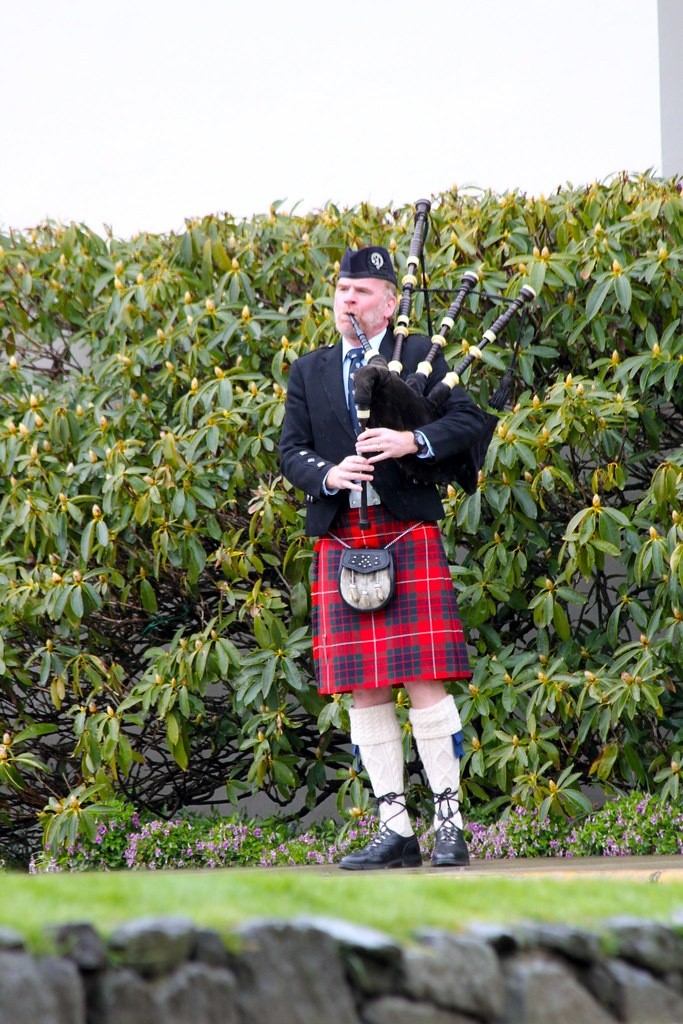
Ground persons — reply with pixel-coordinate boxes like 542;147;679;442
276;245;500;871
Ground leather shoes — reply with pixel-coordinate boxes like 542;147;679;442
429;826;470;868
338;827;422;869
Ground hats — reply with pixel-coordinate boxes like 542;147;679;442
335;246;399;288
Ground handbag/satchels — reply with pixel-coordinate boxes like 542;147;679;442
338;546;395;609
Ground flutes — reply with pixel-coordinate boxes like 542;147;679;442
348;196;537;529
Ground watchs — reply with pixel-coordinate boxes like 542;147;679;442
411;432;425;456
